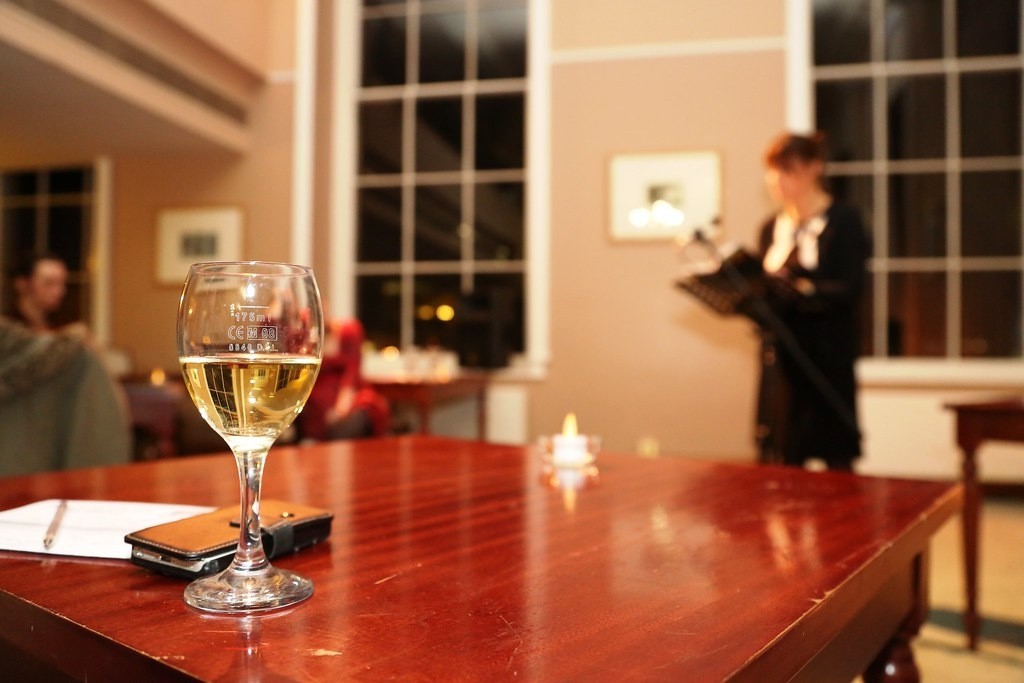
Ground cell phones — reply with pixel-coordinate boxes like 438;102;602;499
125;501;333;581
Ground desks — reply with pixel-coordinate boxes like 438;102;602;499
367;372;491;442
0;431;966;683
943;392;1023;652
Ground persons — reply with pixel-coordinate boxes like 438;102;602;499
753;133;868;474
0;257;65;332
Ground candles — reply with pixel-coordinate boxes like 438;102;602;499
553;411;588;462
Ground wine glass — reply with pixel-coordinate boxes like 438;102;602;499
174;260;329;613
